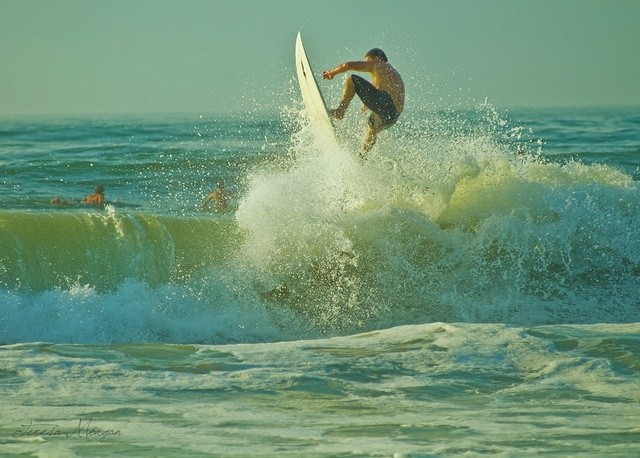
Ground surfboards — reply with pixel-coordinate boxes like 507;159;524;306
295;31;338;143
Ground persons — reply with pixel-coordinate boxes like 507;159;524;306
201;181;241;210
51;183;106;206
324;49;405;167
